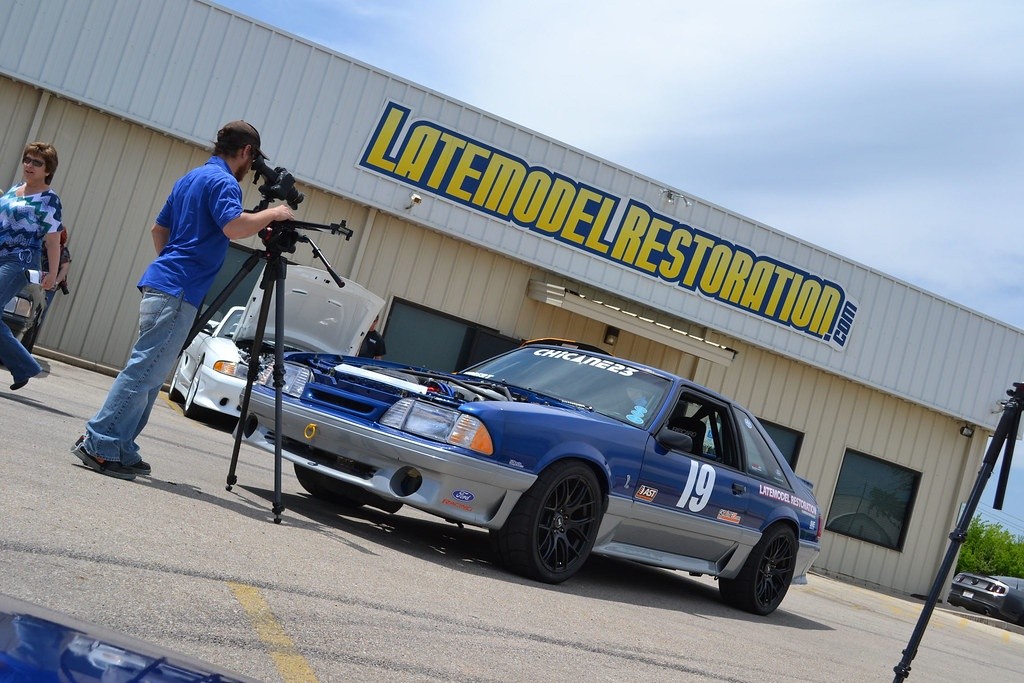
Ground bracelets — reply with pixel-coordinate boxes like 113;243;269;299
55;281;60;285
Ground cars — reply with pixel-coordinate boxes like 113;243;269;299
237;336;824;616
167;260;389;430
947;572;1024;627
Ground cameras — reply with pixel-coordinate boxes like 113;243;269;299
58;281;69;295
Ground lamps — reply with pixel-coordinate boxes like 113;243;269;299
960;421;974;438
658;188;693;208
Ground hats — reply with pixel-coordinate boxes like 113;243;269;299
217;120;271;161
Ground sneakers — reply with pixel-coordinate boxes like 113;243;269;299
118;454;151;476
70;435;136;480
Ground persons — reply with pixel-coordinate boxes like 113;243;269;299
71;122;296;481
0;142;63;390
358;314;387;360
21;224;70;354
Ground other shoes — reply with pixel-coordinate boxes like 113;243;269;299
11;377;29;391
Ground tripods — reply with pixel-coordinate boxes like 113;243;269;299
183;232;345;524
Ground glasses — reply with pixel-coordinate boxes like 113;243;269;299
23;156;47;167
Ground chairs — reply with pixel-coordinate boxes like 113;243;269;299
667;416;706;457
